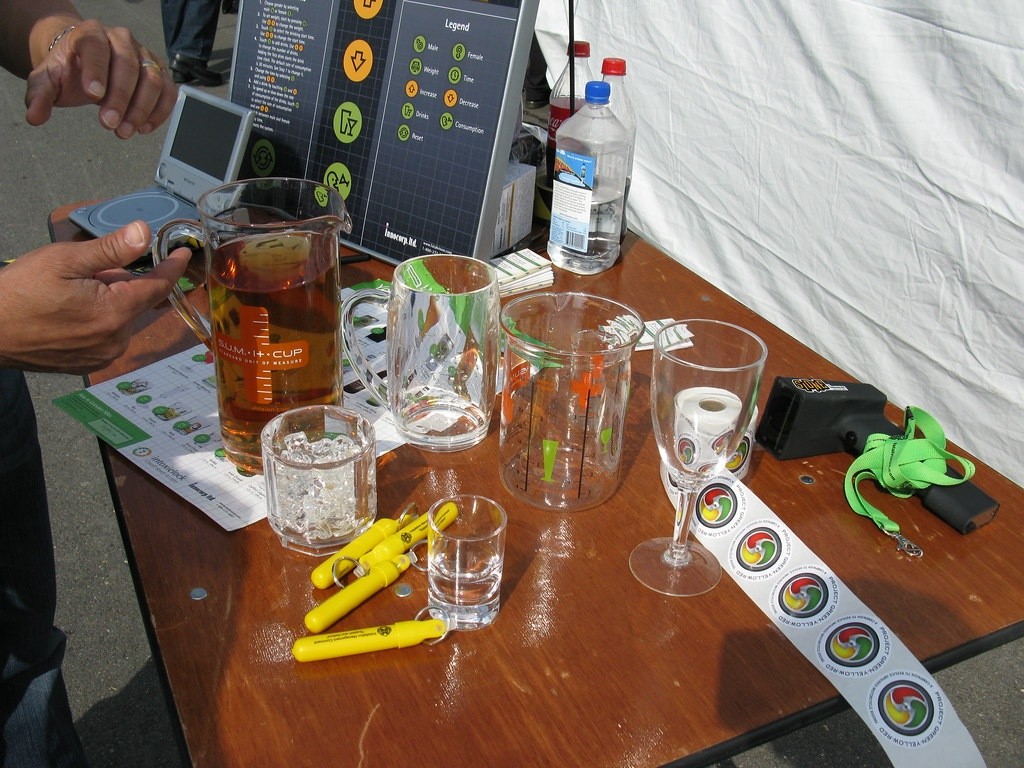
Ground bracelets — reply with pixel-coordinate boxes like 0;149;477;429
47;24;76;51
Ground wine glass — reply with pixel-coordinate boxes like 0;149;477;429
628;319;767;597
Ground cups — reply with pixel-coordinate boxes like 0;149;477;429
261;406;379;556
498;289;644;512
340;254;501;453
427;492;507;632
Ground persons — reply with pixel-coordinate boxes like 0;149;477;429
161;0;224;86
488;0;553;109
0;0;178;768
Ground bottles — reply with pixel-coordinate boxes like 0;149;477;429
547;82;630;275
545;41;593;227
601;58;636;244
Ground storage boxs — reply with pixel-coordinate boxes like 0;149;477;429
490;160;536;257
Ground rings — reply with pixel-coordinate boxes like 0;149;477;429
139;59;160;71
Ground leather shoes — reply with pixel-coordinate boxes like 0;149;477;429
172;70;198;84
169;52;224;86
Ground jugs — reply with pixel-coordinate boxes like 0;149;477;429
152;177;352;477
